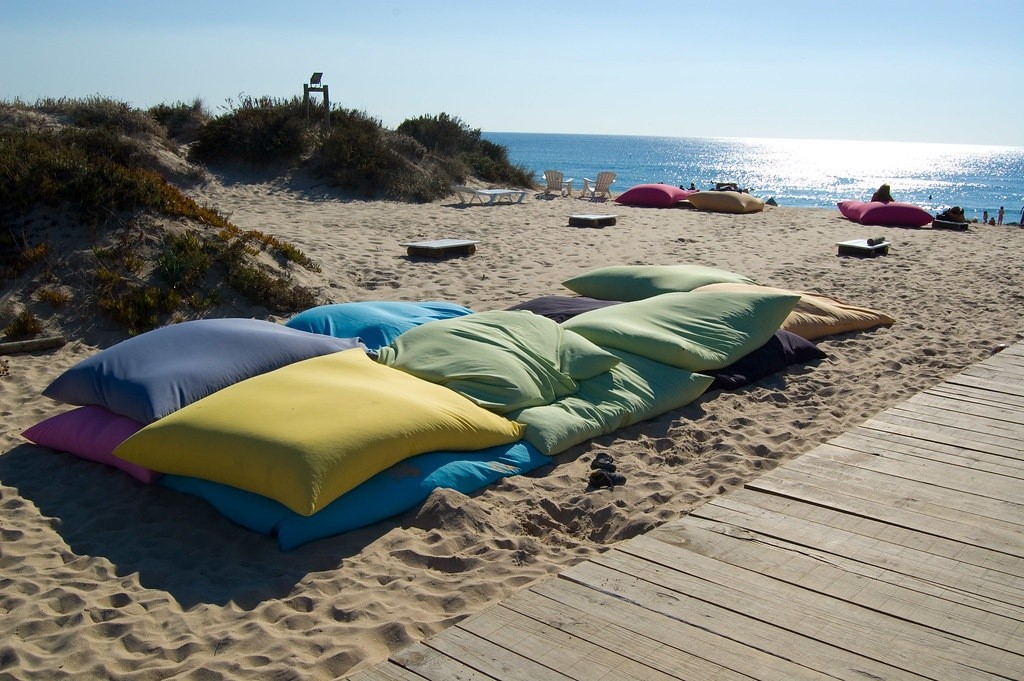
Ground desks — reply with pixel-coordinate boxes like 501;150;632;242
835;238;892;257
399;239;480;260
566;214;618;228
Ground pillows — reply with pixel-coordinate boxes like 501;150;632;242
615;184;700;207
20;263;894;552
837;201;936;226
687;190;764;213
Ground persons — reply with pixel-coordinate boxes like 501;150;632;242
871;184;895;205
657;179;696;192
973;204;1024;228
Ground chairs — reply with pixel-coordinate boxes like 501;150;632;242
450;185;529;207
541;170;574;197
580;171;617;199
